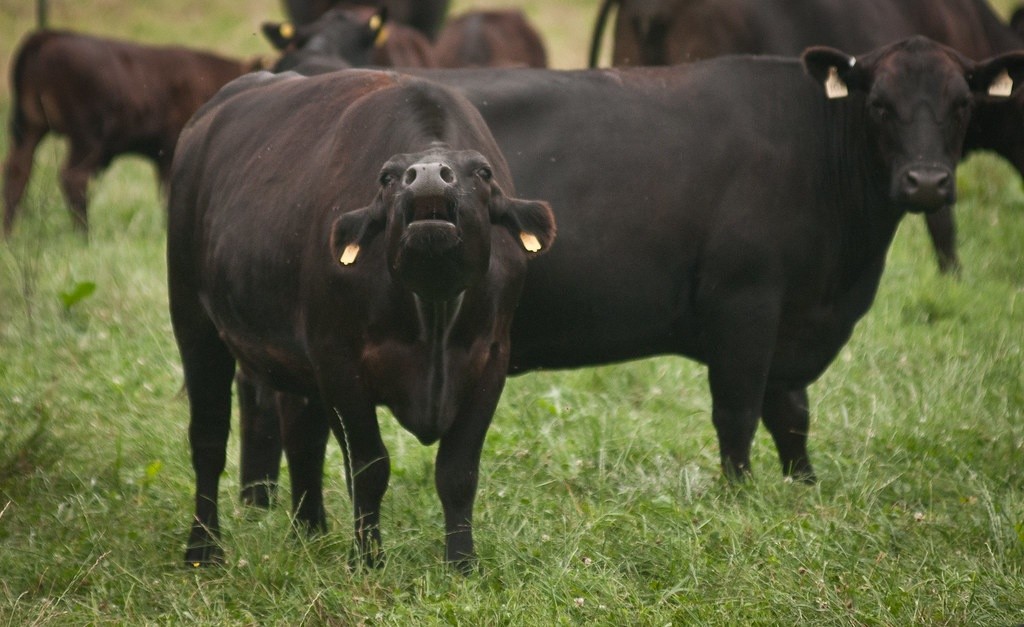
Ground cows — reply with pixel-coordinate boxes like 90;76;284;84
166;69;556;577
239;35;1024;509
0;0;1023;278
